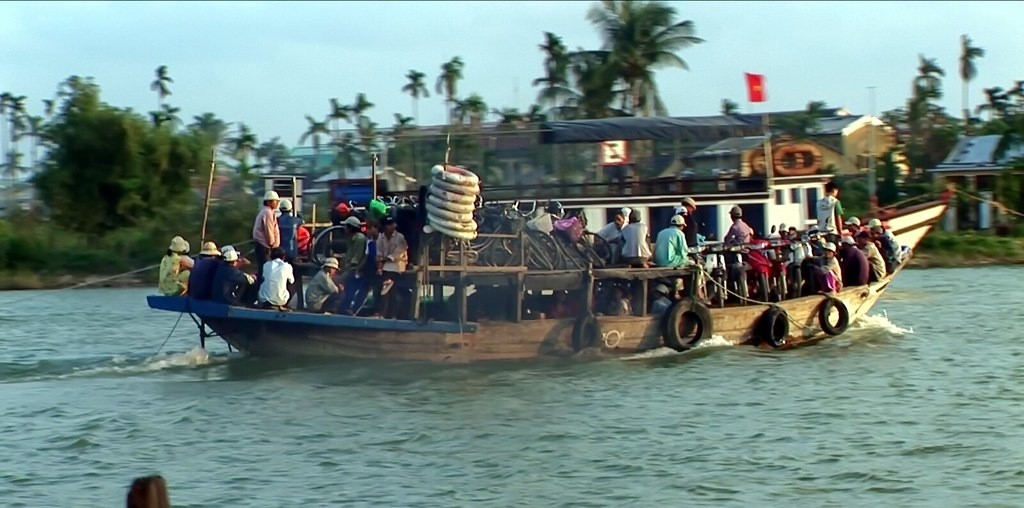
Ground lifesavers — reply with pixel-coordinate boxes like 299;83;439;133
663;297;714;353
429;183;476;203
433;176;481;196
428;193;475;213
426;214;478;231
430;221;478;242
431;164;479;185
819;298;850;335
759;306;790;347
426;201;473;223
572;312;601;352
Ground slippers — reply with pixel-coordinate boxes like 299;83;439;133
381;280;393;295
370;313;384;318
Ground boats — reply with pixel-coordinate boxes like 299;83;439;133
146;50;954;367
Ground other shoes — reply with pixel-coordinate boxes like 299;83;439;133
674;294;683;299
693;294;711;305
278;306;287;311
346;309;354;315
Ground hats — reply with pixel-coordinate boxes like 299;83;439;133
322;257;340;270
279;200;293;211
842;236;858;245
264;190;280;201
729;207;742;217
379;215;396;224
340;216;362;228
823;241;837;255
220;245;241;261
671;198;696;226
656;284;669;295
629;209;641;221
169;235;190;252
864;218;883;234
780;227;790;233
844;217;861;226
199;241;221;255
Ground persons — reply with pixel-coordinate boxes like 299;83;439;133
941;183;957;231
543;182;901;315
158;191;408;319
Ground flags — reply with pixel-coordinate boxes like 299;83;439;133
745;73;765;102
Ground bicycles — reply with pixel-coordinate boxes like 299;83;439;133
427;201;612;271
